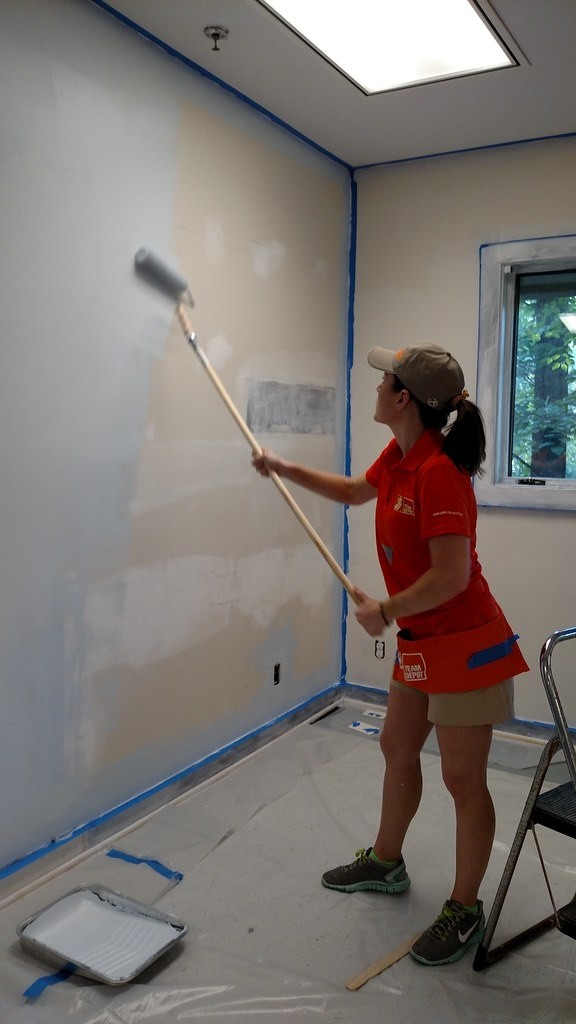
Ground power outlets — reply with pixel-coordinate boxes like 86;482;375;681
375;640;385;659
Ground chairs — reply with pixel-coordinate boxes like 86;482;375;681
471;627;576;974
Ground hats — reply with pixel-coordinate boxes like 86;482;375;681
367;342;465;411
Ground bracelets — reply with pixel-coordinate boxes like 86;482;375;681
378;599;393;627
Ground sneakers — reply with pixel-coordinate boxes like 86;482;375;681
410;899;485;965
321;847;411;893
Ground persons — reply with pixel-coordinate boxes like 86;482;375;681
251;343;533;968
